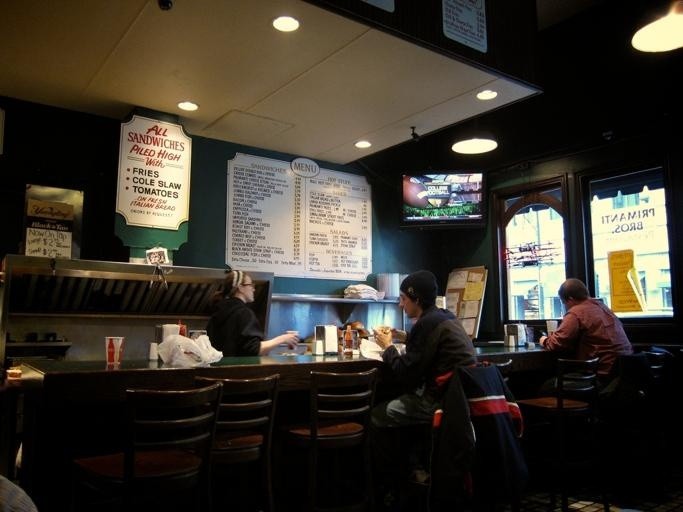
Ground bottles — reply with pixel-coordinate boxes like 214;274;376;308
344;325;353;358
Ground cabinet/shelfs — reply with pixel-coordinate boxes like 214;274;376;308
272;292;405;347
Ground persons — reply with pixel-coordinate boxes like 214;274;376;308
205;271;298;356
370;271;478;429
539;278;633;400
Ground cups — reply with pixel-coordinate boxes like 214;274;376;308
509;335;517;346
546;321;558;332
104;337;125;364
343;330;358;350
312;340;325;356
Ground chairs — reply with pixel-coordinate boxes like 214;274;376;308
516;356;609;512
289;367;377;441
195;373;281;458
595;352;673;416
117;381;222;485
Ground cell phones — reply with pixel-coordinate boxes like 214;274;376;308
539;329;548;338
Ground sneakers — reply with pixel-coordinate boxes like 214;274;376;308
407;464;432;486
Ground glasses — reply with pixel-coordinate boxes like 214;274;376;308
241;282;255;288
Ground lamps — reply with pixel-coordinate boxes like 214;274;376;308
353;139;372;149
631;1;683;53
177;100;200;112
451;128;498;155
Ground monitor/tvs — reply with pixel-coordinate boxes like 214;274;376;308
396;165;490;227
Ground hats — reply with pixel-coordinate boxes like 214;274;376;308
400;271;437;305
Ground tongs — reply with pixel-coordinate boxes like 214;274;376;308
150;264;168;289
